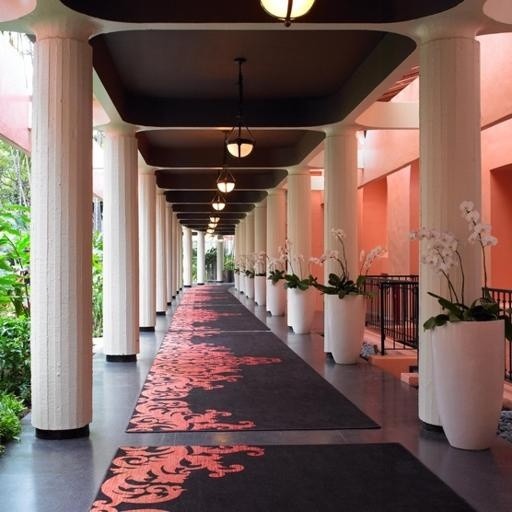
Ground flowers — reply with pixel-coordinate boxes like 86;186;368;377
412;200;511;332
237;227;322;290
323;227;389;299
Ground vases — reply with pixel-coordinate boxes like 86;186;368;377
429;318;505;451
232;270;289;317
283;278;321;335
319;286;372;364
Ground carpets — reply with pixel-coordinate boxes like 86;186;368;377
90;283;476;511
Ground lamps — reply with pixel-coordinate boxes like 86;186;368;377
259;0;316;27
224;57;257;159
206;161;236;234
